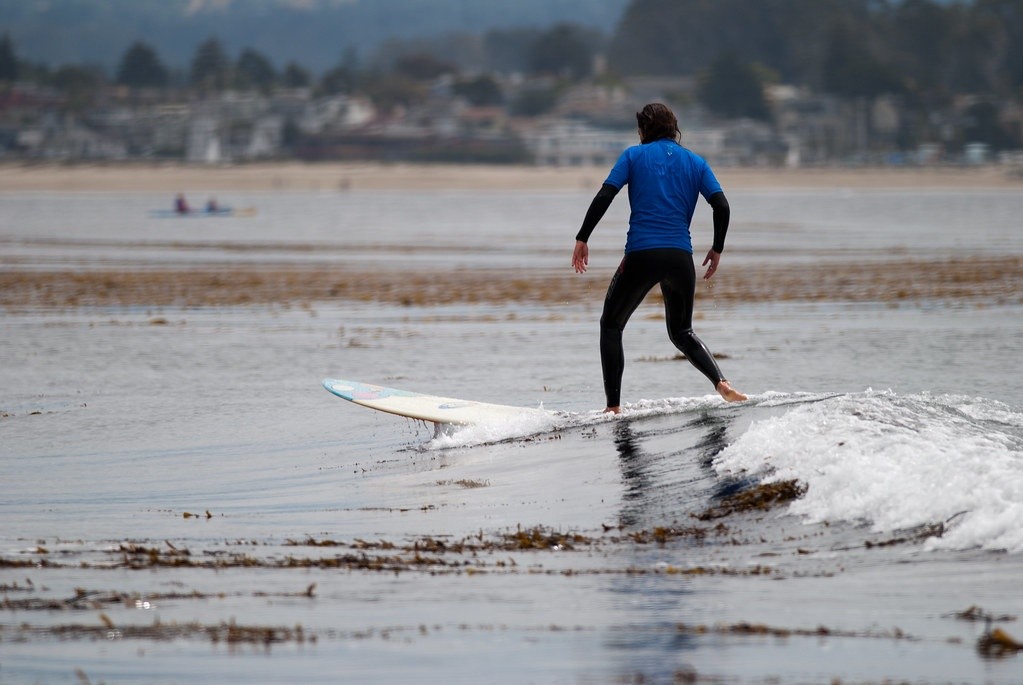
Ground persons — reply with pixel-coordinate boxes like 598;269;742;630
207;200;216;213
177;194;187;212
571;103;748;414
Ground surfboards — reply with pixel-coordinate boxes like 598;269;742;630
318;374;847;436
